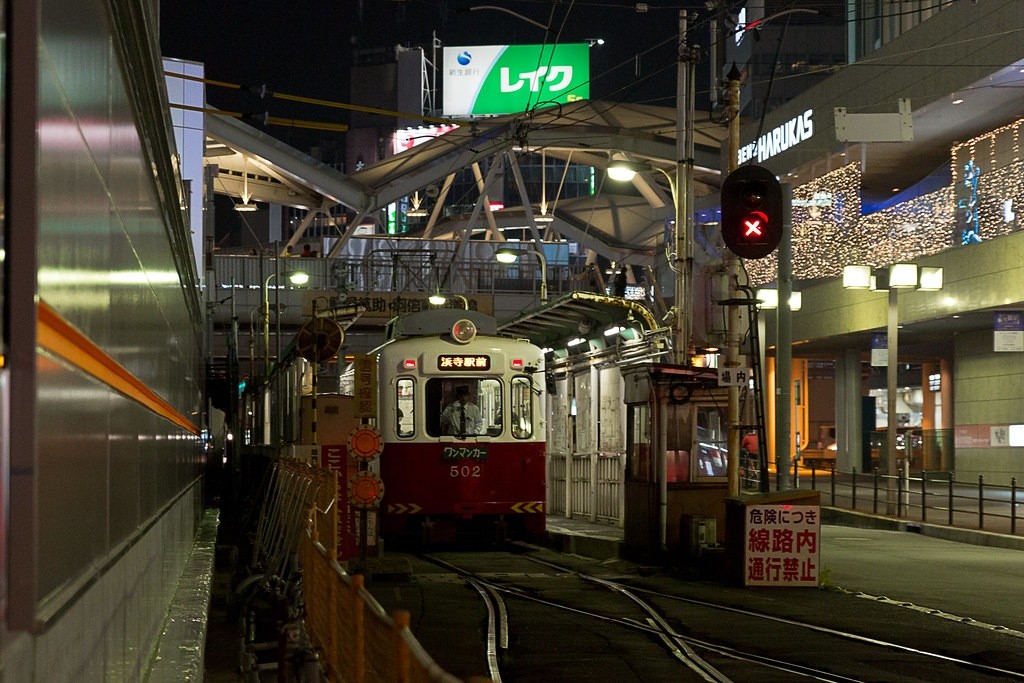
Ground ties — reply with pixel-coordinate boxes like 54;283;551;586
459;406;467;434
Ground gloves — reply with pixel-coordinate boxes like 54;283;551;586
452;400;461;412
473;429;479;435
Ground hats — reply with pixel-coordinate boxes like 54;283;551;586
453;384;471;396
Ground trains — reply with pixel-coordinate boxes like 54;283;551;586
337;307;549;551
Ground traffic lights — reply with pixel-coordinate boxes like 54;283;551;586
720;164;783;260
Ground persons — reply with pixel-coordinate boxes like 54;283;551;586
614;266;627;298
280;245;294;258
309;251;317;258
587;263;600;294
576;266;588;291
440;384;484;436
299;243;312;257
398;408;410;438
740;431;759;471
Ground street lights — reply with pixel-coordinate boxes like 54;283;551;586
264;270;312;374
250;303;283;382
430;292;469;312
495;247;550;305
606;159;688;367
842;263;943;515
